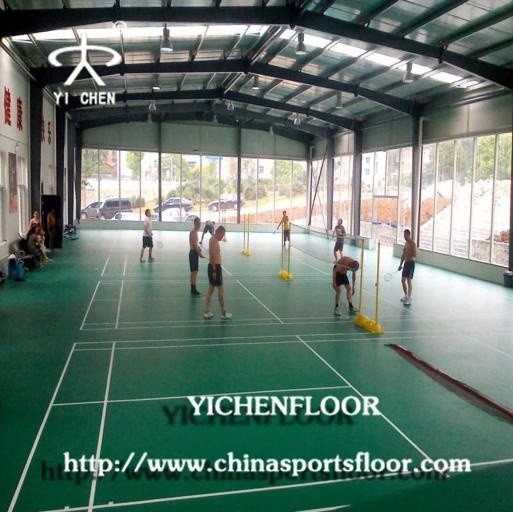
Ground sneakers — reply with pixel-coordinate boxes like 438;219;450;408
348;307;358;313
334;308;342;316
191;288;200;294
203;312;214;318
221;313;233;319
401;297;412;305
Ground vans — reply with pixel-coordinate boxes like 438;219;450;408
80;198;133;220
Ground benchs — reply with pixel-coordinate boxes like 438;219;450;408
11;239;35;272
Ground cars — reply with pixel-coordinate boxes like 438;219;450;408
209;194;245;212
153;197;192;214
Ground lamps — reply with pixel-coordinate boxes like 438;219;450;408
403;62;414;83
295;32;307;57
160;28;173;54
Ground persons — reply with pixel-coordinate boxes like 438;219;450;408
332;256;359;316
397;229;417;305
276;210;290;247
188;217;205;294
202;226;232;319
139;208;156;263
198;220;215;244
331;219;346;264
24;206;56;270
